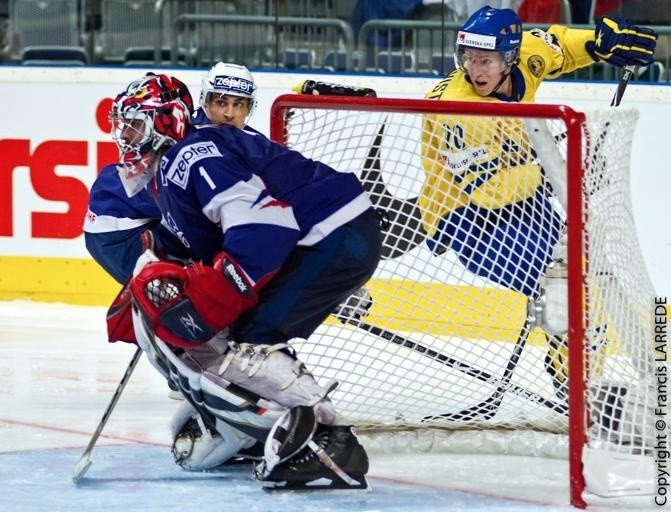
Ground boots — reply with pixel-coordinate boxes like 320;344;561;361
251;425;371;481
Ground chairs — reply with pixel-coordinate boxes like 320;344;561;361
6;0;339;68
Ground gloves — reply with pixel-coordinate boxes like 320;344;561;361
586;18;662;71
134;251;263;350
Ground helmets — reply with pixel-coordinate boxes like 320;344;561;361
199;61;260;129
455;5;522;75
112;72;193;145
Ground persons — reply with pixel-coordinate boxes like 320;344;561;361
416;4;671;455
79;59;261;390
106;69;385;489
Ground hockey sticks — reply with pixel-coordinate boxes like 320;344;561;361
73;346;143;482
422;69;633;422
335;311;626;434
